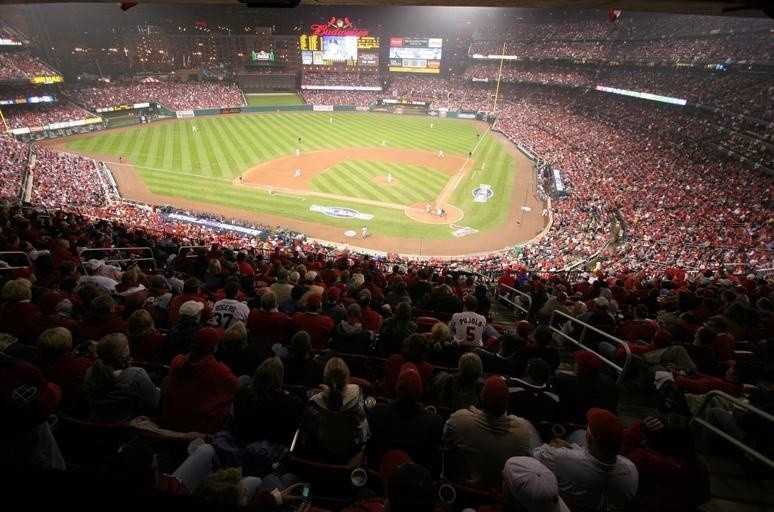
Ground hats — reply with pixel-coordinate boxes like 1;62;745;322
502;454;560;511
458;352;483;381
178;299;205;318
506;260;774;401
479;375;510;417
394;362;424;402
585;406;625;456
183;238;480;315
86;258;106;270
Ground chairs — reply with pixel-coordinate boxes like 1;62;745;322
0;214;774;512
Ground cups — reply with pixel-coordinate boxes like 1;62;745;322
552;424;566;436
438;486;456;504
365;398;377;408
425;406;437;414
187;439;205;456
350;469;368;488
